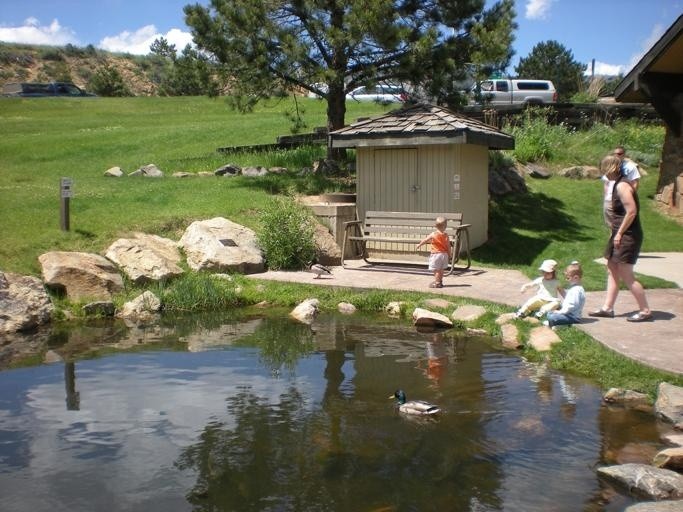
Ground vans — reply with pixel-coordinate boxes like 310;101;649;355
344;83;407;102
460;78;558;107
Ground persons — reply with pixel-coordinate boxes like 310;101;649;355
515;259;567;318
416;216;451;287
583;384;642;510
546;263;587;327
587;155;653;321
511;359;553;407
602;146;640;203
557;374;581;419
422;337;446;395
481;83;493;92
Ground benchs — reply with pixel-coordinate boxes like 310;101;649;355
341;211;472;277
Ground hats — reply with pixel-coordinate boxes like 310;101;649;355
538;258;558;273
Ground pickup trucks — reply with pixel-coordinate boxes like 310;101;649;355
0;82;96;97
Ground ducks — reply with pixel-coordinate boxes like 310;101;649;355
308;258;334;279
388;389;442;416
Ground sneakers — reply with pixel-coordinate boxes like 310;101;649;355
626;311;654;322
428;279;443;289
588;307;614;318
